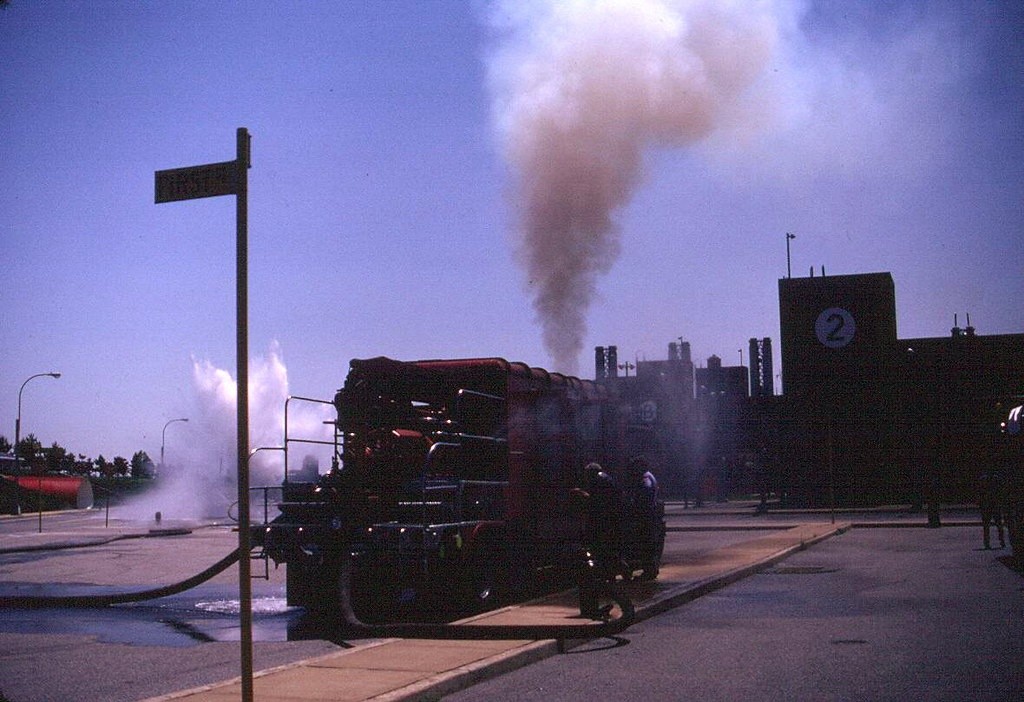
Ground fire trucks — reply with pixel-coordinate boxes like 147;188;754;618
252;358;636;595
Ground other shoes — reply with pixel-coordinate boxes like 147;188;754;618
635;572;656;582
617;612;636;625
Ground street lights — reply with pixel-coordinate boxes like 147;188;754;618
13;372;61;514
160;418;190;476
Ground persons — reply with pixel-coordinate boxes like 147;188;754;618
978;462;1024;566
922;465;941;529
569;462;635;626
